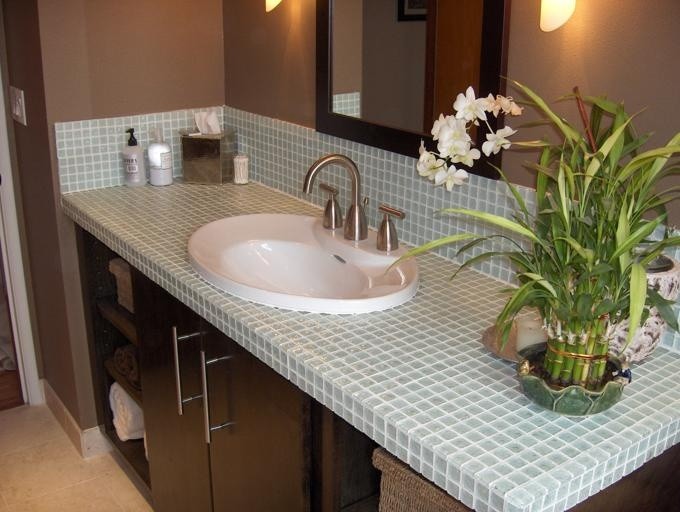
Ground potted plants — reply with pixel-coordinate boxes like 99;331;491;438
381;70;679;417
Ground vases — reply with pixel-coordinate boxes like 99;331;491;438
563;246;679;363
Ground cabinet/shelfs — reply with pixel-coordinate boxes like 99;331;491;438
78;218;154;512
318;409;680;512
135;275;318;512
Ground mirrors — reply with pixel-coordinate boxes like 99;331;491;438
317;0;511;184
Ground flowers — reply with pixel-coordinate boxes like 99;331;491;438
408;78;680;274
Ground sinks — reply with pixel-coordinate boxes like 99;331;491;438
186;213;418;316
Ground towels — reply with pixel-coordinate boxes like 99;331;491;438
109;342;139;389
105;384;140;443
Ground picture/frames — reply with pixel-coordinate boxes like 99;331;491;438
394;0;429;22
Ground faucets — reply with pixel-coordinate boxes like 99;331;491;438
303;153;370;241
